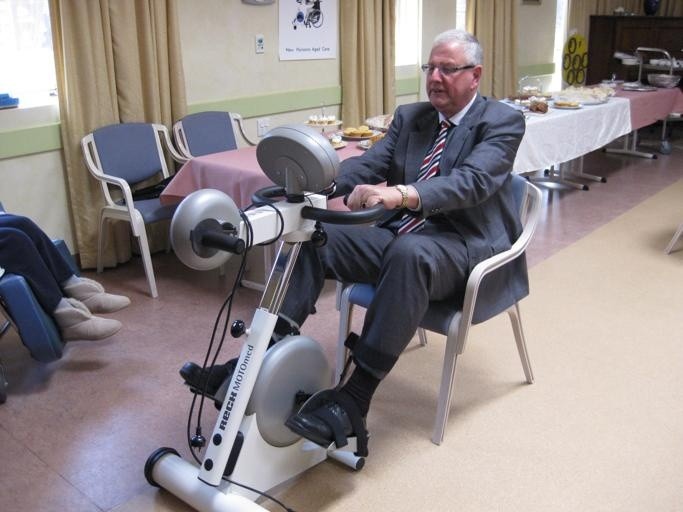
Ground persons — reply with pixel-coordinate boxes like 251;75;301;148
177;26;530;450
0;214;131;342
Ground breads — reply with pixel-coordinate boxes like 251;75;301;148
344;125;373;137
530;102;548;113
310;119;337;124
554;102;578;106
370;133;384;144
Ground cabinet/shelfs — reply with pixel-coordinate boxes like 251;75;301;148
586;15;683;89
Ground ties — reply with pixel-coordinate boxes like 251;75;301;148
397;119;451;233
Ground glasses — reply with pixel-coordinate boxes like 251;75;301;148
422;64;475;75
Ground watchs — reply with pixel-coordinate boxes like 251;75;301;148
396;184;407;210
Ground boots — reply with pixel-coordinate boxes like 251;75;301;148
63;278;130;314
53;298;121;341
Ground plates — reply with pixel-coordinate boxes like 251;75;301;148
334;143;346;150
335;130;381;140
357;140;371;149
550;105;584;109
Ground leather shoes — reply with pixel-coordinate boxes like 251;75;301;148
180;358;239;396
285;400;367;448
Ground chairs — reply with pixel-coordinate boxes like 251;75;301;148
81;124;226;294
0;202;92;409
334;172;545;446
172;112;277;271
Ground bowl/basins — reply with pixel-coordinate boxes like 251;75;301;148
647;73;681;89
